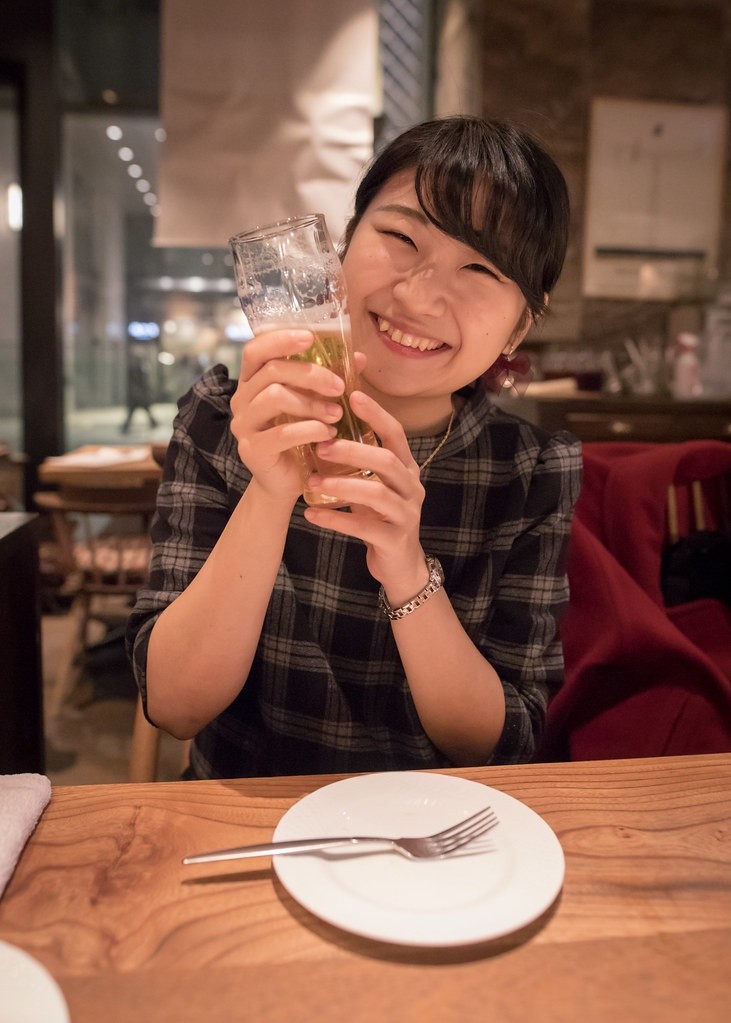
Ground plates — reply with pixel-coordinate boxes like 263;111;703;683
270;771;567;948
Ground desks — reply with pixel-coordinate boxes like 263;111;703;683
33;484;159;708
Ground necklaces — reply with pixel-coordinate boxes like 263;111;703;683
417;406;456;475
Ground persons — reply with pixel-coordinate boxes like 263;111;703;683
121;343;158;430
119;115;590;787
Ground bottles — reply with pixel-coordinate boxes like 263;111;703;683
670;332;704;404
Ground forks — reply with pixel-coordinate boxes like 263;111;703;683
182;806;500;864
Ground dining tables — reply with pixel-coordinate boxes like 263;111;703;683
0;749;731;1023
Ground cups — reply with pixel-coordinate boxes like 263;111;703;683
230;212;381;514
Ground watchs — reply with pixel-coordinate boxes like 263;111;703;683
377;555;445;620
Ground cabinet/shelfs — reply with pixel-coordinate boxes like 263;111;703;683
491;392;730;445
0;510;46;777
